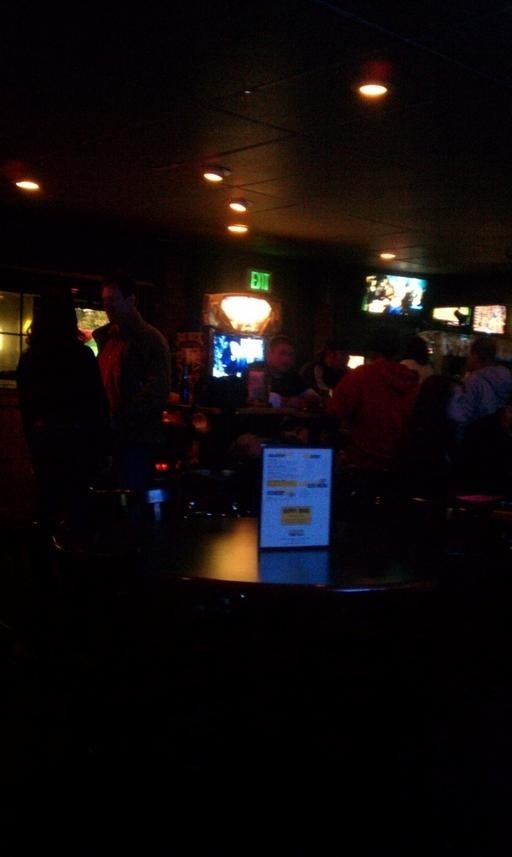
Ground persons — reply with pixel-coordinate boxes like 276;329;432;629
17;271;512;544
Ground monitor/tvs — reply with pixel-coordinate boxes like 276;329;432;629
208;331;266;379
471;304;507;335
359;272;429;321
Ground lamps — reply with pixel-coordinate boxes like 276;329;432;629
357;56;392;100
228;223;249;234
229;199;248;213
380;253;396;260
202;165;226;182
15;180;39;190
